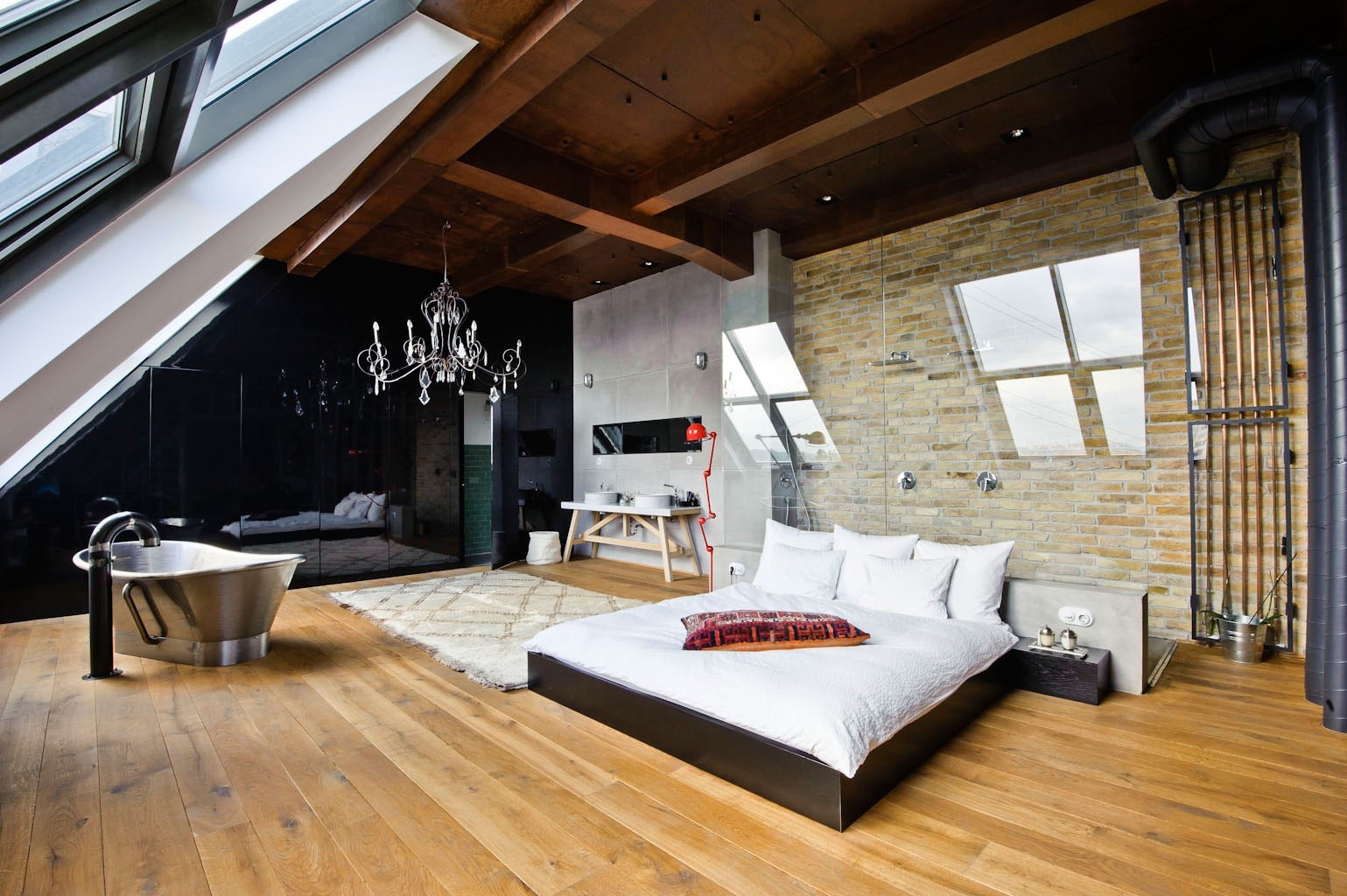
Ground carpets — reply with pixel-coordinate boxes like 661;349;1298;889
240;537;454;577
330;570;650;691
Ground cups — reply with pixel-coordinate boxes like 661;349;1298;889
690;498;698;506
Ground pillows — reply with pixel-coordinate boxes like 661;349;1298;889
335;492;387;521
681;519;1015;649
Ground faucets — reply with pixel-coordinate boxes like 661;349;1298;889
599;482;604;492
81;510;163;680
662;483;678;506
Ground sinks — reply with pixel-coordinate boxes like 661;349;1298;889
584;491;618;503
634;494;671;506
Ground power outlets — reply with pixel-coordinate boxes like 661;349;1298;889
1059;607;1093;627
729;563;745;575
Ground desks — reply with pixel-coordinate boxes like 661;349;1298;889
561;501;701;582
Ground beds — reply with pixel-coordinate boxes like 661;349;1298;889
224;512;387;544
520;516;1017;832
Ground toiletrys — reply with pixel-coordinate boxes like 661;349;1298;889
619;490;640;504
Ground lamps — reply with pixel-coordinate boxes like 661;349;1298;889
694;352;705;369
584;374;593;387
357;221;526;404
686;423;717;591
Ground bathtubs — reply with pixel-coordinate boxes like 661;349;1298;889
70;538;308;667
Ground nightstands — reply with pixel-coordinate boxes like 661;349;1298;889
1005;637;1110;706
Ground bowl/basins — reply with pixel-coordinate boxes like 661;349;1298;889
679;501;689;507
